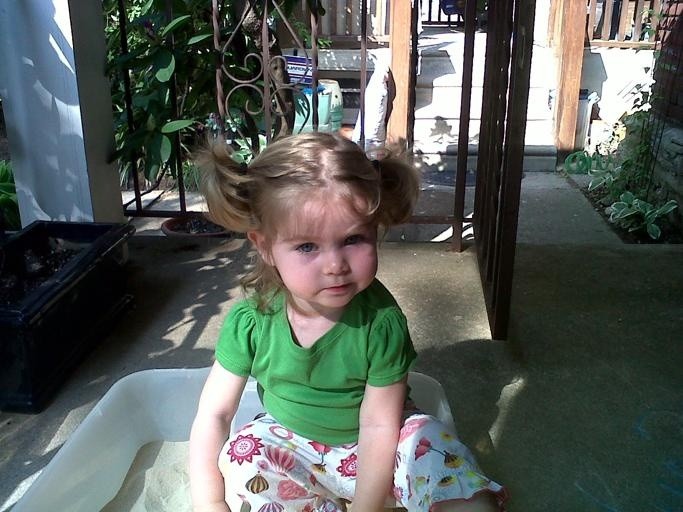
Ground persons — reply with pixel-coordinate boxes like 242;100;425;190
188;130;509;512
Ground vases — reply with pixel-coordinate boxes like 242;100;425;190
161;212;232;237
